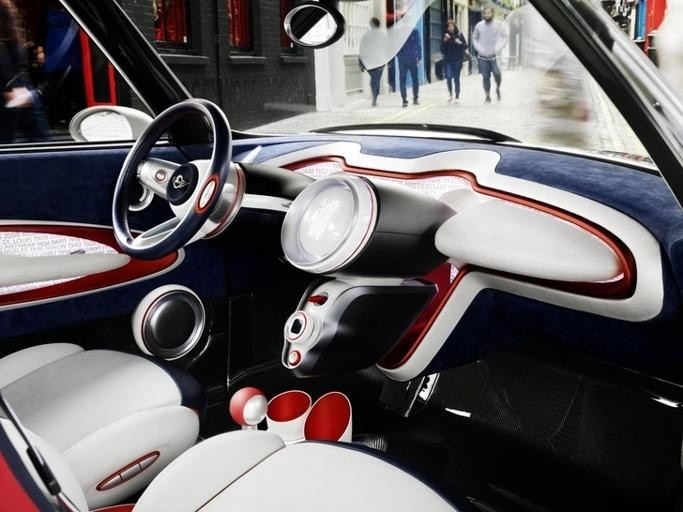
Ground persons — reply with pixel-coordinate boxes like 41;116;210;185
471;6;509;103
395;12;422;107
357;16;387;106
440;19;467;104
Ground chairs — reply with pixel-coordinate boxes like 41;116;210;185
0;339;208;512
129;430;462;512
